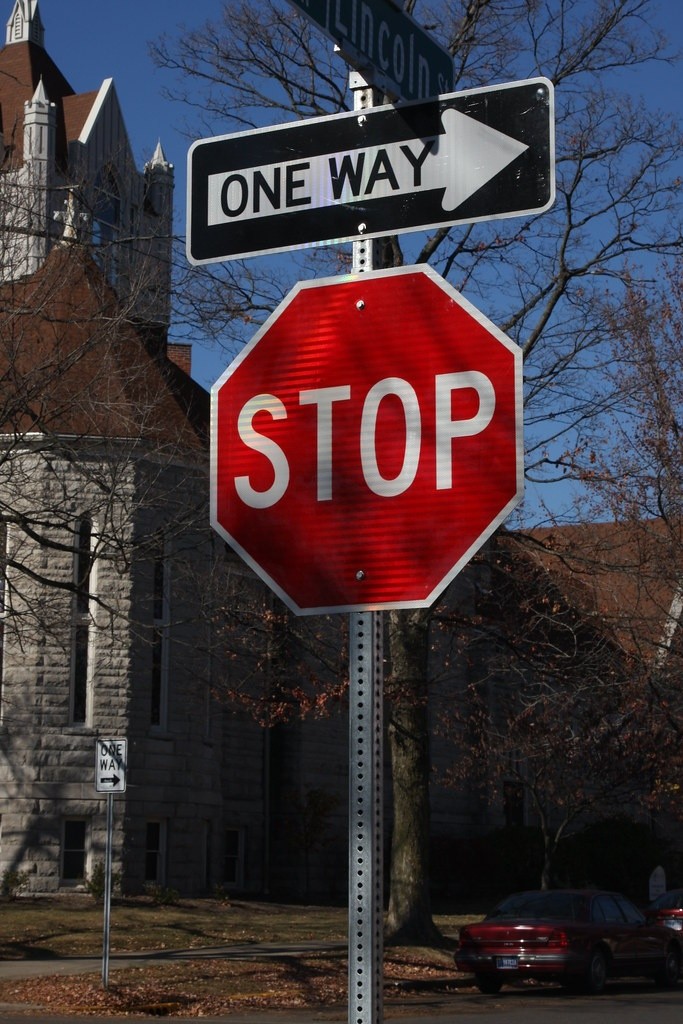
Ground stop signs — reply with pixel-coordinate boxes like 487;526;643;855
208;264;524;618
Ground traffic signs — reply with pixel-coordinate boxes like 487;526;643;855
93;738;129;793
187;73;558;264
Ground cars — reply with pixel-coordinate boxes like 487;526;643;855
645;888;683;939
452;888;683;1000
